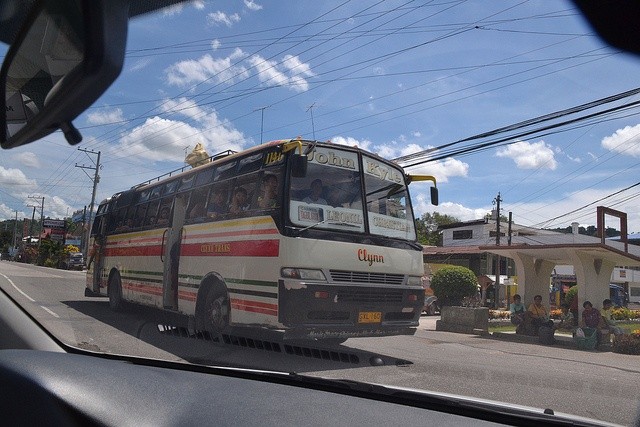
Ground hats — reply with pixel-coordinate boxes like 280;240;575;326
603;299;611;305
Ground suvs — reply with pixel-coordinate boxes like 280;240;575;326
610;284;628;307
63;253;84;271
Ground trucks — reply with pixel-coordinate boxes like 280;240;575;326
423;276;439;316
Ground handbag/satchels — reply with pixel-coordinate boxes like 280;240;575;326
533;302;549;324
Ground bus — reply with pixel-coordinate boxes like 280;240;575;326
85;135;438;343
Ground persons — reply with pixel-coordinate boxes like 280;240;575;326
115;222;124;231
599;298;625;337
104;223;111;233
553;303;574;330
125;219;135;229
302;178;328;205
256;175;280;208
327;190;344;208
188;200;206;218
527;295;555;336
510;294;527;335
156;207;173;226
147;216;155;225
580;300;602;341
205;191;229;220
228;188;251;215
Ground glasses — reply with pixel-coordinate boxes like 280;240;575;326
535;298;541;301
